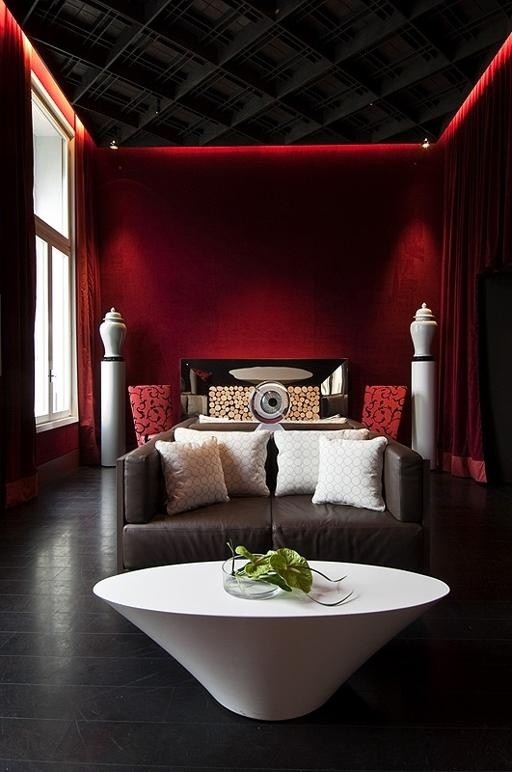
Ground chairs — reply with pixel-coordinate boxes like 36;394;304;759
361;384;407;443
127;384;182;449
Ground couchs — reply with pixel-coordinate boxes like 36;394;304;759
116;415;428;571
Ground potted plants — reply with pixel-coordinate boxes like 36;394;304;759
219;541;355;608
